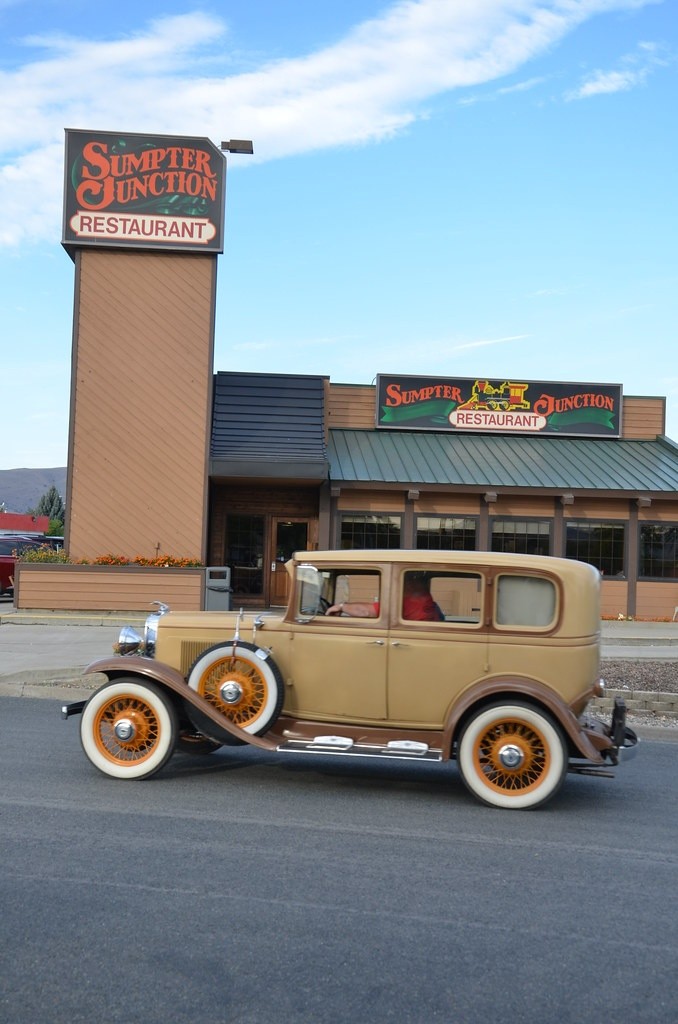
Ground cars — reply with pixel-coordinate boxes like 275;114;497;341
0;538;53;597
60;549;638;810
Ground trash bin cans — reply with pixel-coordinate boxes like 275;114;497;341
205;566;231;611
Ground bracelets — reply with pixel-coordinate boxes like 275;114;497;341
339;602;347;611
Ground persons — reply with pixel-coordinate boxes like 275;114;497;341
325;573;444;622
